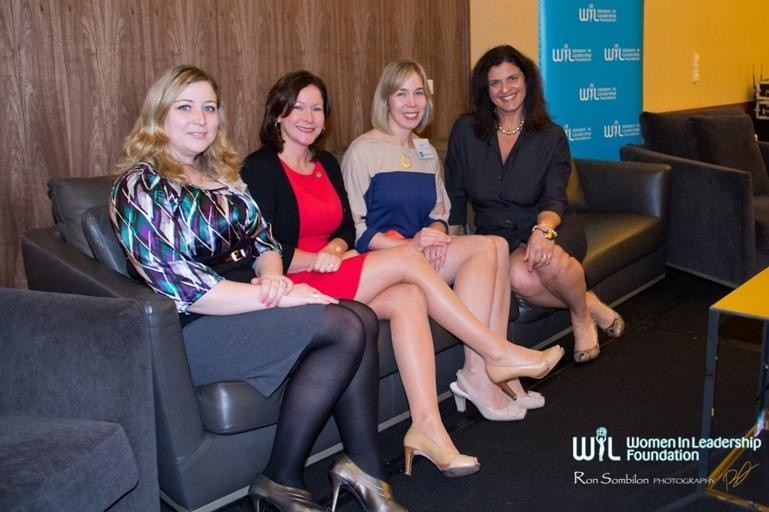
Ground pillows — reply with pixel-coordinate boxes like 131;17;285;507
689;117;769;196
566;161;590;212
51;177;113;258
84;203;129;279
640;106;746;160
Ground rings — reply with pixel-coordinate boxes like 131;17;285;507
330;263;335;268
311;291;319;297
279;279;285;283
435;252;441;256
543;255;548;259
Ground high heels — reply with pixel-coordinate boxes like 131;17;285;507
246;473;326;512
486;342;565;401
326;453;411;512
402;423;481;479
453;367;528;423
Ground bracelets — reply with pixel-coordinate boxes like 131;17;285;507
532;224;558;239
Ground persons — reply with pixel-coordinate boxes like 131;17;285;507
107;63;409;512
340;57;547;421
443;44;626;363
240;70;565;478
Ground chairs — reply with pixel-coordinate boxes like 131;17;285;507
0;285;160;512
620;142;769;289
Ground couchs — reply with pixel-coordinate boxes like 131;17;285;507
22;160;673;512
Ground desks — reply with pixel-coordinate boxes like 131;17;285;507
698;266;769;512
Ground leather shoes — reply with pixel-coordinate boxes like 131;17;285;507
584;289;625;339
504;372;546;410
571;314;601;363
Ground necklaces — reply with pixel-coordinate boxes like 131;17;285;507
495;109;527;135
385;131;414;168
